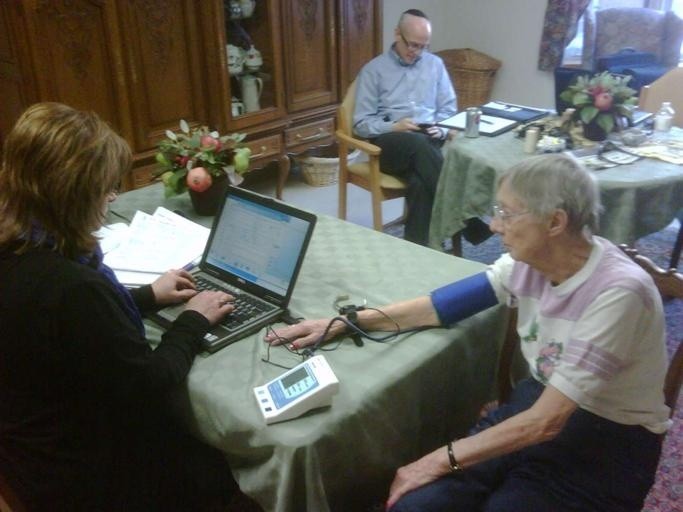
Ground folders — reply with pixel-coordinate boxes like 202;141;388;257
435;99;548;137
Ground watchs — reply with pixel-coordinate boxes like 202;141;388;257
444;436;464;475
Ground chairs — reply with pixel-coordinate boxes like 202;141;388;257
642;65;682;129
481;243;683;444
582;7;682;83
336;75;462;257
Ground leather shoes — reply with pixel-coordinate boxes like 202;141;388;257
461;217;495;246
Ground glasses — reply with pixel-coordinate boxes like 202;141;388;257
491;204;534;223
399;32;431;55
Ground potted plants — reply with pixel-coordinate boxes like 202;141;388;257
558;73;642;143
142;119;254;214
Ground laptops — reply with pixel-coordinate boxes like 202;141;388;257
146;184;318;354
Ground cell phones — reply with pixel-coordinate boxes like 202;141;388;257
413;120;435;134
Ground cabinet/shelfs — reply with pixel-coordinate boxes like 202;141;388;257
0;1;384;200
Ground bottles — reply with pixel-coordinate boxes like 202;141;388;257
652;102;674;151
466;107;482;138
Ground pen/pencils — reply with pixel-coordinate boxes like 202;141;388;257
479;119;491;123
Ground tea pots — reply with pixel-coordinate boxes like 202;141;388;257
226;45;246;74
229;96;244;117
234;74;262;113
244;45;263;70
239;1;255;18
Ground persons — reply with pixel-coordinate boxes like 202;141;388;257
262;148;675;510
349;7;495;247
0;100;262;510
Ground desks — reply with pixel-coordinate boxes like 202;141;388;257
94;175;518;512
